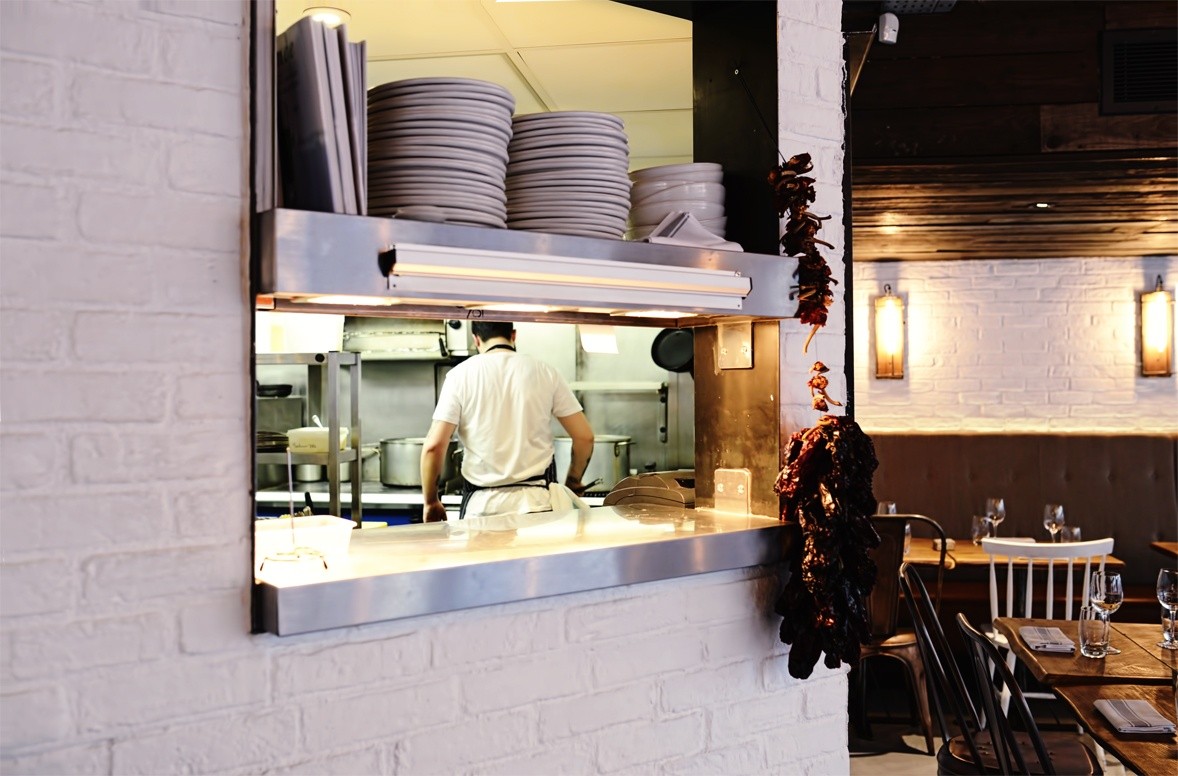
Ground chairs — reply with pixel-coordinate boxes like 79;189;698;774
850;513;1114;776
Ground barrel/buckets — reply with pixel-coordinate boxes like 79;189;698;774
297;447;349;482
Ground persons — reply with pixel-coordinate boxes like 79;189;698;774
421;322;595;524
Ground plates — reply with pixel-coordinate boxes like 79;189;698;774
507;111;633;240
368;76;515;229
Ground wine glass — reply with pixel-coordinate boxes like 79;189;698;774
1091;572;1123;654
987;498;1005;541
1043;504;1064;543
1157;568;1178;649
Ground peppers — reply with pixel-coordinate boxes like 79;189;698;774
765;152;883;680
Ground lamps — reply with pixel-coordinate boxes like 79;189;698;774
377;243;753;312
875;284;905;380
1139;274;1172;379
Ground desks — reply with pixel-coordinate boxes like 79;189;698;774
903;534;1178;776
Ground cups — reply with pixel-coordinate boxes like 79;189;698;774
903;524;912;554
1079;606;1110;658
1161;606;1178;640
877;502;897;515
1061;526;1081;543
972;516;989;547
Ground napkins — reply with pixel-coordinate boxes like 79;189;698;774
1017;626;1077;652
1093;699;1176;734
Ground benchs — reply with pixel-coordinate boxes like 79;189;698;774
896;580;1163;603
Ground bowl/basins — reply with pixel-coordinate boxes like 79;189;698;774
287;427;348;452
626;162;727;242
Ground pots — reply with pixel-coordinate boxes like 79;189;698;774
380;438;463;487
255;378;293;396
651;328;694;373
555;435;638;491
